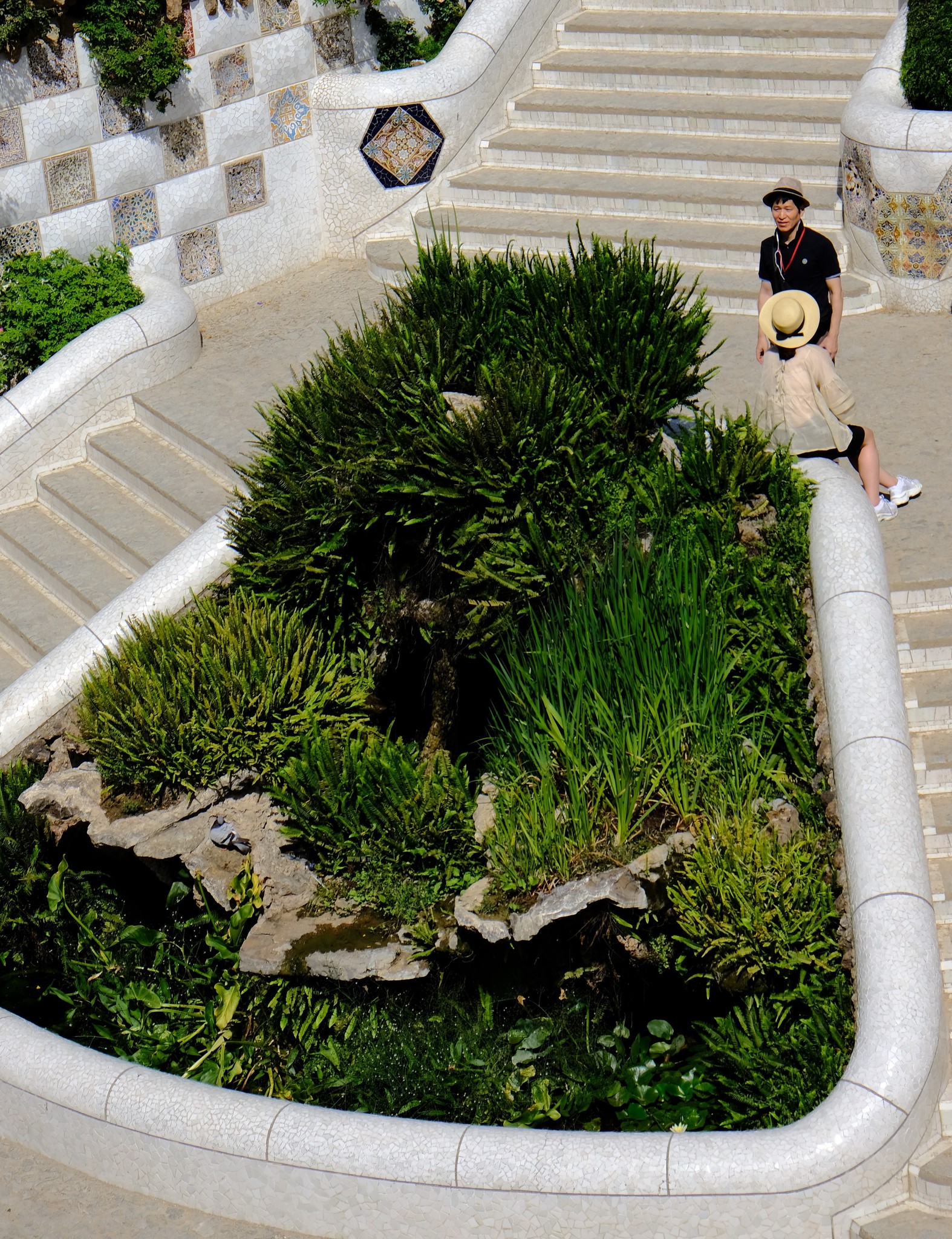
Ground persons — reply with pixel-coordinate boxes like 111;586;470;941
755;176;844;367
751;289;922;523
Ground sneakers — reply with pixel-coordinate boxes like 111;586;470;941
876;494;898;521
889;475;923;506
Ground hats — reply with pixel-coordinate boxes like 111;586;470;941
762;177;810;209
759;290;820;349
